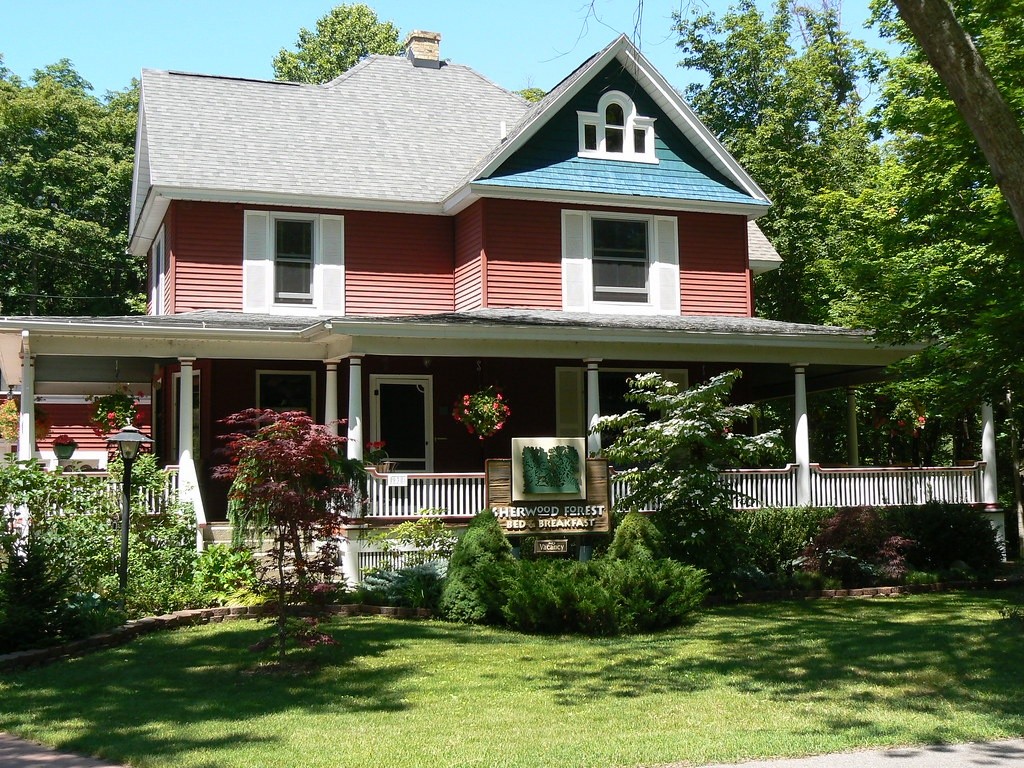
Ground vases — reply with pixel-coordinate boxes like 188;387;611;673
53;444;75;459
366;465;388;472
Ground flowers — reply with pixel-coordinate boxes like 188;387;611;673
450;387;512;440
363;441;387;464
52;434;79;449
82;386;146;432
